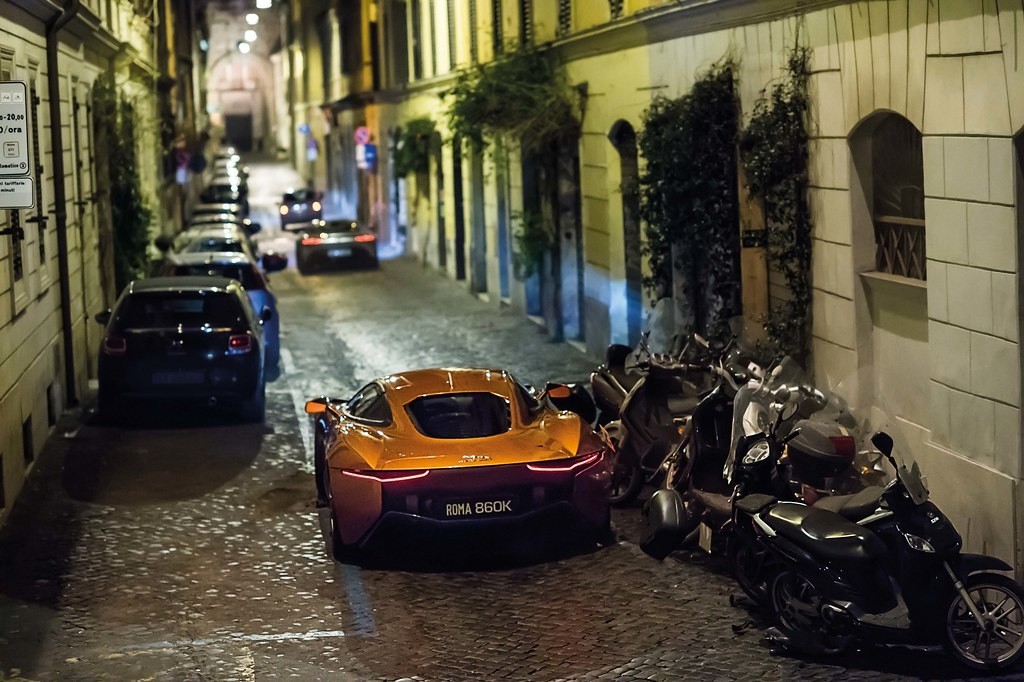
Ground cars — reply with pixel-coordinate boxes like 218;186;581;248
94;276;273;423
275;190;324;230
155;148;261;266
296;219;377;274
151;251;288;368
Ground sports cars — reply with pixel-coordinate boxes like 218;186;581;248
304;367;615;561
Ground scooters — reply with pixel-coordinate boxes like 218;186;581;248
589;330;1024;678
